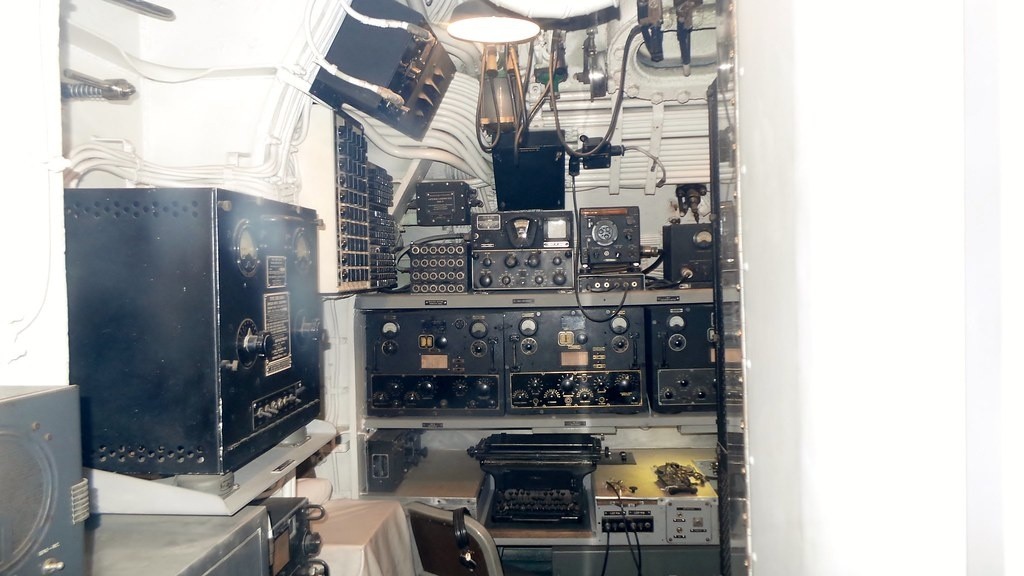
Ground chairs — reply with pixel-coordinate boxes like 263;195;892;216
402;501;504;576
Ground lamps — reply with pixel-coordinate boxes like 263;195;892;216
447;0;541;44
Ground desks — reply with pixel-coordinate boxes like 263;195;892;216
309;498;415;576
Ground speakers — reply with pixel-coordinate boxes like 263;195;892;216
0;384;86;576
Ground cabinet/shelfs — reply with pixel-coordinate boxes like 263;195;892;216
354;286;722;576
